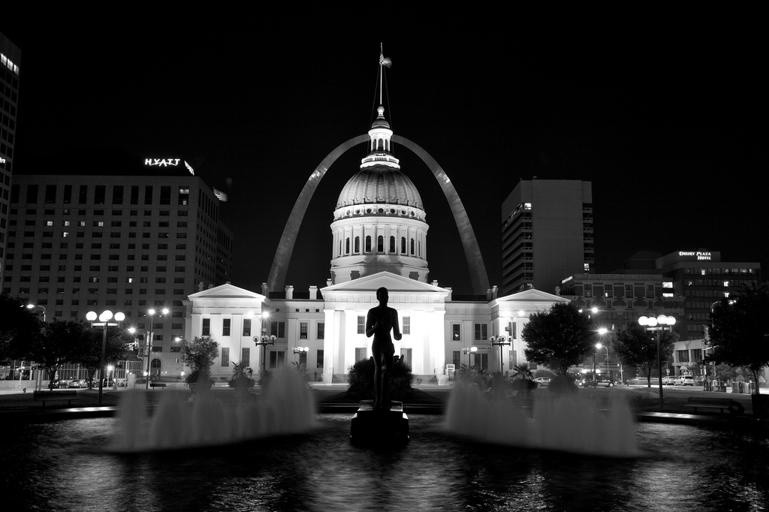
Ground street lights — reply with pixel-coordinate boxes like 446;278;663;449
462;346;478;369
172;333;198;386
85;308;124;406
26;301;49;326
144;305;170;389
636;311;679;409
291;342;311;371
510;310;525;366
489;330;514;375
252;325;278;374
574;299;625;392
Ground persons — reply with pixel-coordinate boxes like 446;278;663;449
365;287;402;409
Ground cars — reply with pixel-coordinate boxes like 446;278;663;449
48;375;127;386
661;375;694;386
533;376;552;387
579;375;616;388
